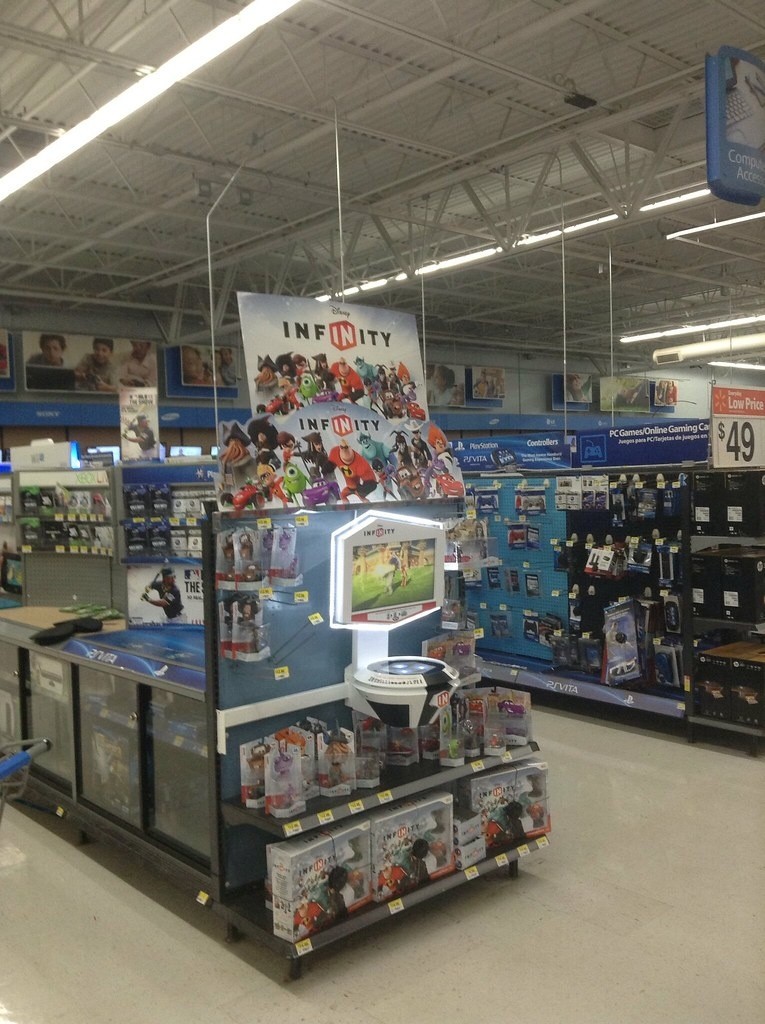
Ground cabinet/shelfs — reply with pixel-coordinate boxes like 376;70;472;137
1;441;551;982
461;463;765;747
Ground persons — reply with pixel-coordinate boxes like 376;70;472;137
426;365;504;406
123;414;156;458
142;567;187;624
26;334;236;393
566;374;677;406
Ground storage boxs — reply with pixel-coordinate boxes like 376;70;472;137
265;758;550;942
691;543;765;623
697;639;764;728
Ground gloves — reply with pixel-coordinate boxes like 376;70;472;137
141;585;150;601
121;433;127;440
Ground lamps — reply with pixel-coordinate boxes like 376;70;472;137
0;1;300;201
351;655;461;728
314;180;710;303
619;313;765;345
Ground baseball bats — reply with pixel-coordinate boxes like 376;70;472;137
122;403;146;439
140;558;170;601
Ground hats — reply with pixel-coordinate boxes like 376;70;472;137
136;414;151;423
161;567;176;577
